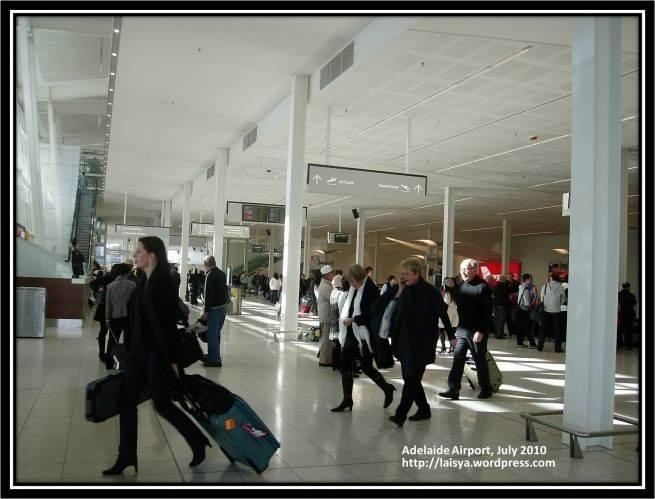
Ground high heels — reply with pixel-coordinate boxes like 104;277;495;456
102;454;139;476
374;372;397;408
188;436;213;467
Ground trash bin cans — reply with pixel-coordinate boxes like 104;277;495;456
227;286;243;315
15;286;47;338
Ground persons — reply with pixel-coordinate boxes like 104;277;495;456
64;235;639;478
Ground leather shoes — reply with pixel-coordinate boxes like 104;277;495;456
409;408;431;421
389;414;404;428
478;391;492;399
439;390;460;400
203;361;222;368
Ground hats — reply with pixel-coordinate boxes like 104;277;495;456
320;265;332;274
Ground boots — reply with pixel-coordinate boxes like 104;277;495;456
331;380;354;413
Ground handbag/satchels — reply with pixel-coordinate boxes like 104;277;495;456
512;303;520;324
378;297;402;339
171;325;204;374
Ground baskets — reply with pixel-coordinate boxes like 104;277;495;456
84;367;153;423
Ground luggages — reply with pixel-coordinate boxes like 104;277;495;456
462;344;504;394
168;361;281;477
